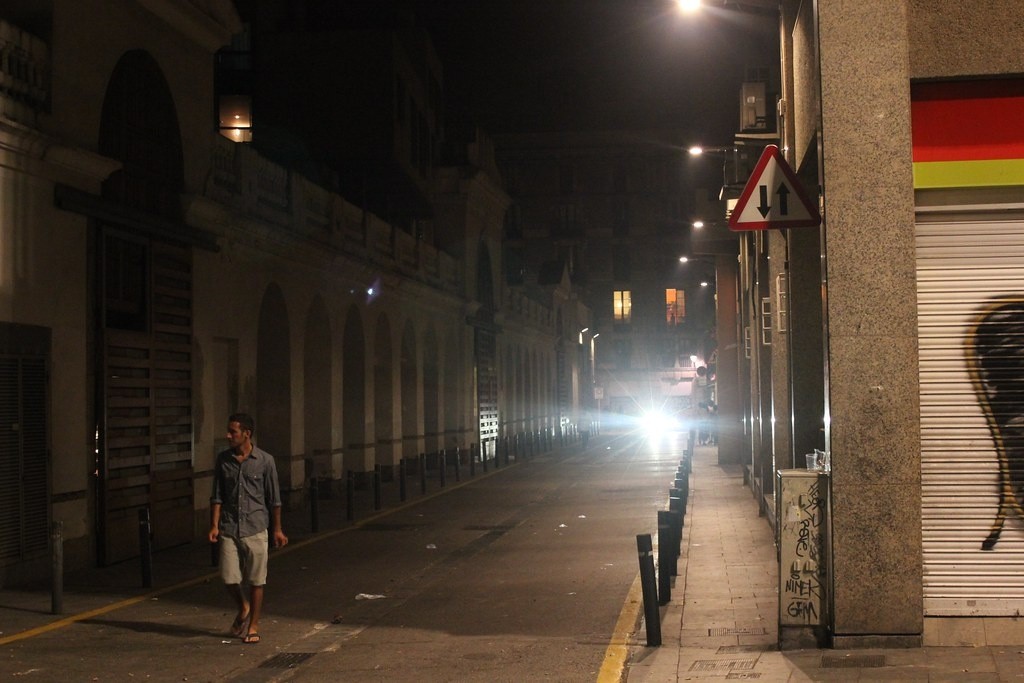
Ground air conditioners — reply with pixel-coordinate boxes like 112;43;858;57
740;80;767;132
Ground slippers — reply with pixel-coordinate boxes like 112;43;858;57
245;633;261;643
231;611;250;637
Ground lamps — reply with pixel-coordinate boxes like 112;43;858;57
219;94;255;144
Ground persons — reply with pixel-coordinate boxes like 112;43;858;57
670;312;675;328
698;400;719;447
208;413;288;643
579;410;592;450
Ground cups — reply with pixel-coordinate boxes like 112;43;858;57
805;454;818;471
